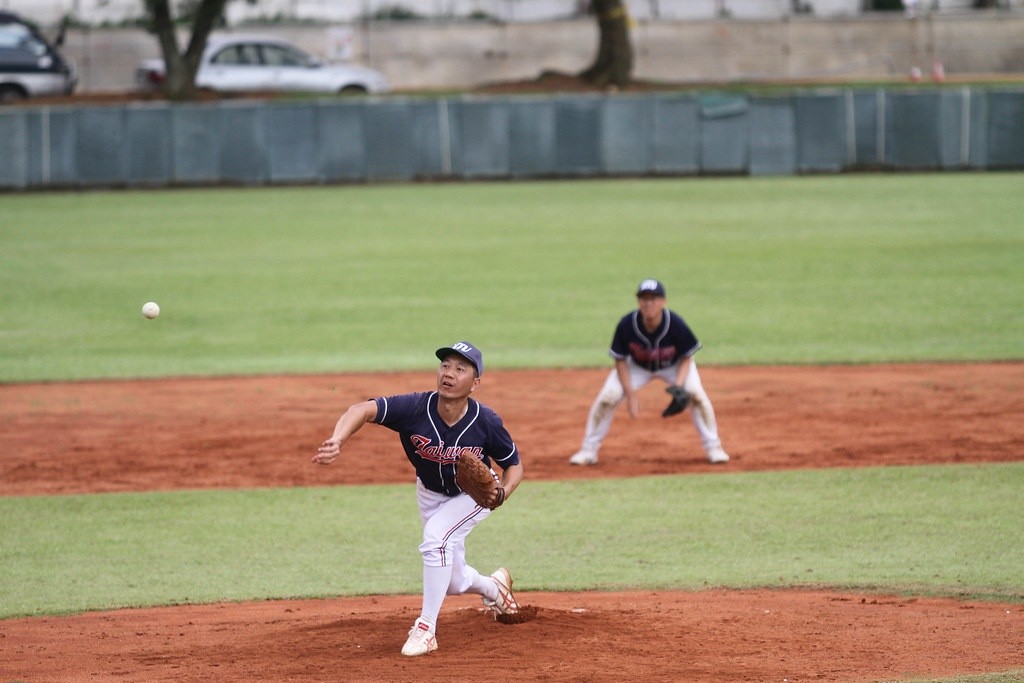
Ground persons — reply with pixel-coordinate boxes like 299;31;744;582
310;341;523;657
568;278;730;465
902;1;948;87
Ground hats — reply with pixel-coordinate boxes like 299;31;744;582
435;341;483;378
636;279;665;298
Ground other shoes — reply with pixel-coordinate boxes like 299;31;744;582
569;448;598;466
706;446;730;463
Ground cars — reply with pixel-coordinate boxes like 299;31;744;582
132;31;391;98
0;9;79;101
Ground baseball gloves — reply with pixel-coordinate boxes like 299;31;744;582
662;385;691;417
455;449;506;512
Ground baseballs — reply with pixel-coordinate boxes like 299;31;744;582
142;301;160;319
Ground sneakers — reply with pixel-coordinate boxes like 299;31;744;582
482;566;520;621
400;618;438;656
